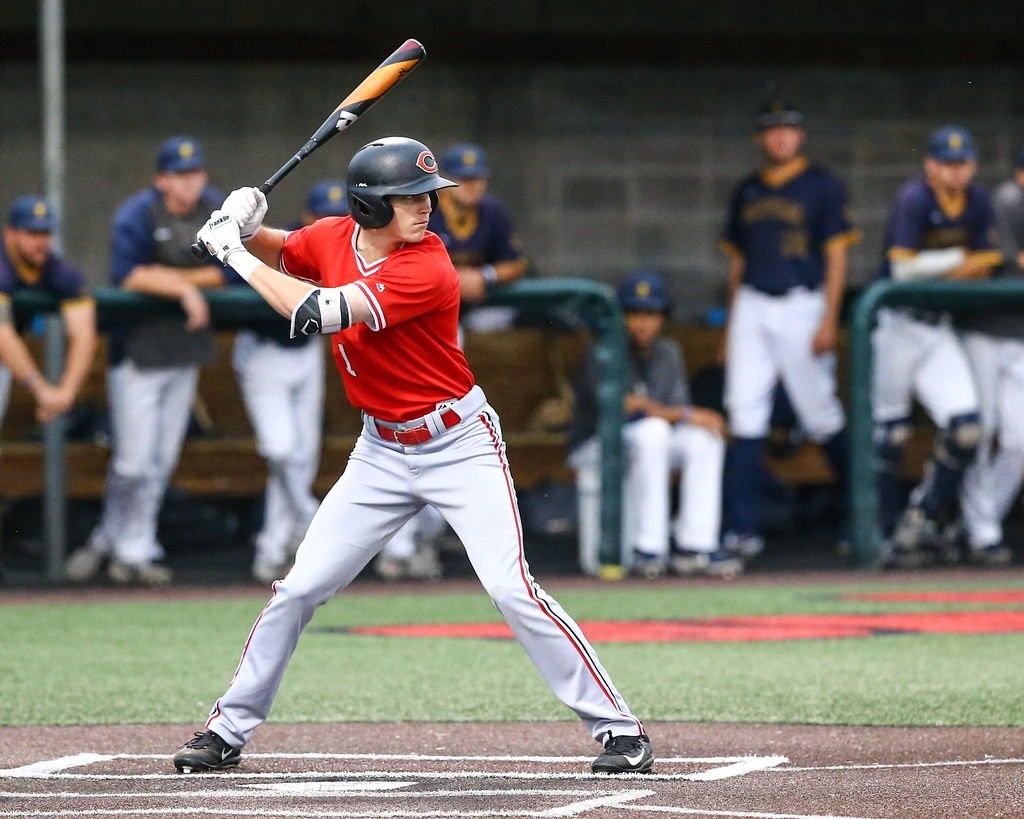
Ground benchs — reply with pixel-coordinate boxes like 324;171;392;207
0;318;1022;503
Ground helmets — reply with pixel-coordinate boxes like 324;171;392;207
346;135;459;231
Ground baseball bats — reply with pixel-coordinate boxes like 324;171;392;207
189;36;429;260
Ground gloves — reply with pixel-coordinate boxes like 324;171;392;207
196;186;268;263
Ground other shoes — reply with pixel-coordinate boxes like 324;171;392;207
971;543;1014;566
375;539;448;584
64;539;103;582
106;559;177;588
631;550;668;580
671;546;745;578
249;557;283;586
878;535;939;569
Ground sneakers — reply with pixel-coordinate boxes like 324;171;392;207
591;730;655;772
173;730;241;773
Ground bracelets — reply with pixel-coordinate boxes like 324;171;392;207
479;261;501;288
677;404;693;424
18;371;42;391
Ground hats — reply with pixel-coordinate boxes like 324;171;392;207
304;181;349;216
619;274;673;317
922;124;975;162
441;143;493;180
6;195;58;231
156;136;206;175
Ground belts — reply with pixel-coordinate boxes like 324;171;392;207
375;409;461;446
893;306;941;325
752;282;817;298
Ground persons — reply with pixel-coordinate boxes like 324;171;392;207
63;141;238;590
0;195;96;434
706;101;887;576
375;141;534;581
564;277;744;579
869;122;1024;569
174;136;654;772
231;182;350;588
